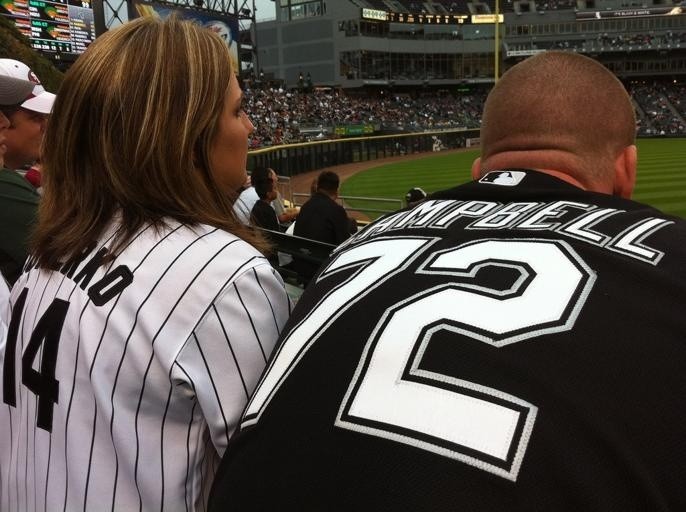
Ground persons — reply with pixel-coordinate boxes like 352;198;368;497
5;2;684;512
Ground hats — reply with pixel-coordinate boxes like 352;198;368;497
0;59;57;114
405;187;427;202
